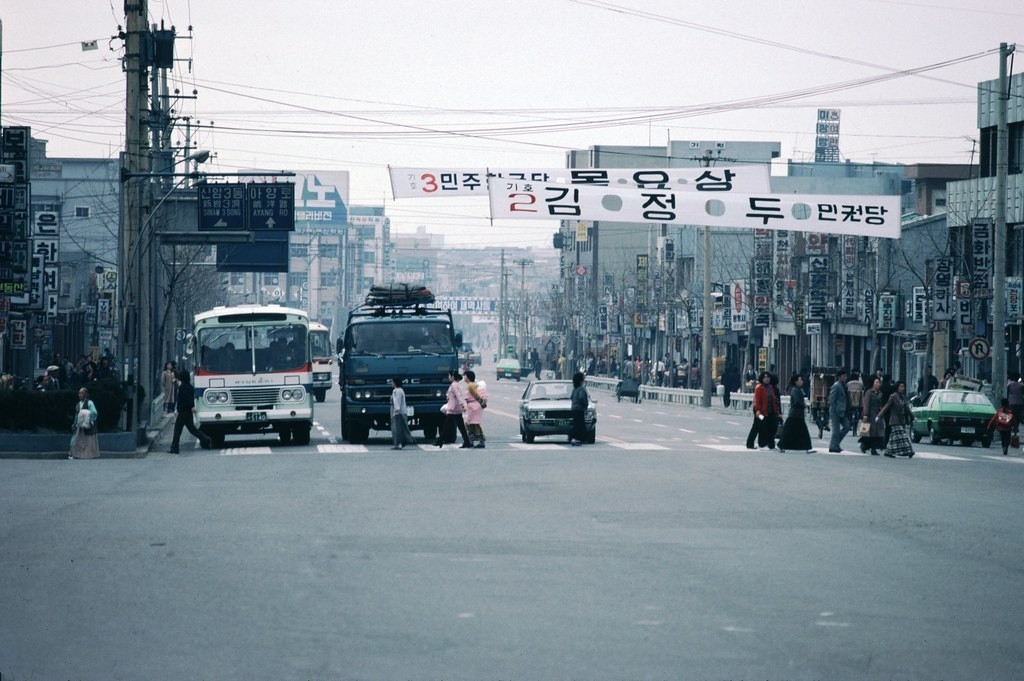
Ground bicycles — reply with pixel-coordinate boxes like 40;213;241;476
814;408;829;439
849;409;859;437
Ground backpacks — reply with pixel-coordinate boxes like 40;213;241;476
998;409;1014;426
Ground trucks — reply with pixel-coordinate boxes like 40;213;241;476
335;300;462;445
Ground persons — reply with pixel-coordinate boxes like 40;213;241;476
167;371;212;454
811;367;914;466
69;387;98;459
918;361;964;396
390;376;417;449
375;329;399;354
19;348;114;433
568;372;588;445
527;347;566;380
775;374;816;454
462;371;487;448
721;362;740;408
576;352;700;390
746;372;778;450
1007;372;1024;433
987;398;1014;455
162;362;176;413
218;343;242;373
744;364;756;409
421;327;445;354
270;337;296;371
431;370;473;448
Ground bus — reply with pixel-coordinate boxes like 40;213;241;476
307;322;333;403
184;303;314;449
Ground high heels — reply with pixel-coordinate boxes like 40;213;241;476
459;444;471;448
433;442;443;448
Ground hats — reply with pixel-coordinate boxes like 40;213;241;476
47;366;59;371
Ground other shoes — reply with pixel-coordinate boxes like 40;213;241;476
749;447;757;449
775;445;785;453
871;452;879;455
168;449;178;453
884;453;895;458
859;443;865;453
206;438;212;451
909;452;915;458
806;450;816;453
475;444;485;448
392;447;402;450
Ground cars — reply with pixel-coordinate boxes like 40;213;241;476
457;341;482;369
908;389;998;448
496;359;521;381
518;378;598;444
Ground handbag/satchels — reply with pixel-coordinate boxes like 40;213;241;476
1011;433;1019;447
858;419;871;437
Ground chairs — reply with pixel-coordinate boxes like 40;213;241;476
536;386;546;395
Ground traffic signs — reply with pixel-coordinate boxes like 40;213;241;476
197;183;248;232
247;183;296;232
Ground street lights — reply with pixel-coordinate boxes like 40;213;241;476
117;149;212;433
